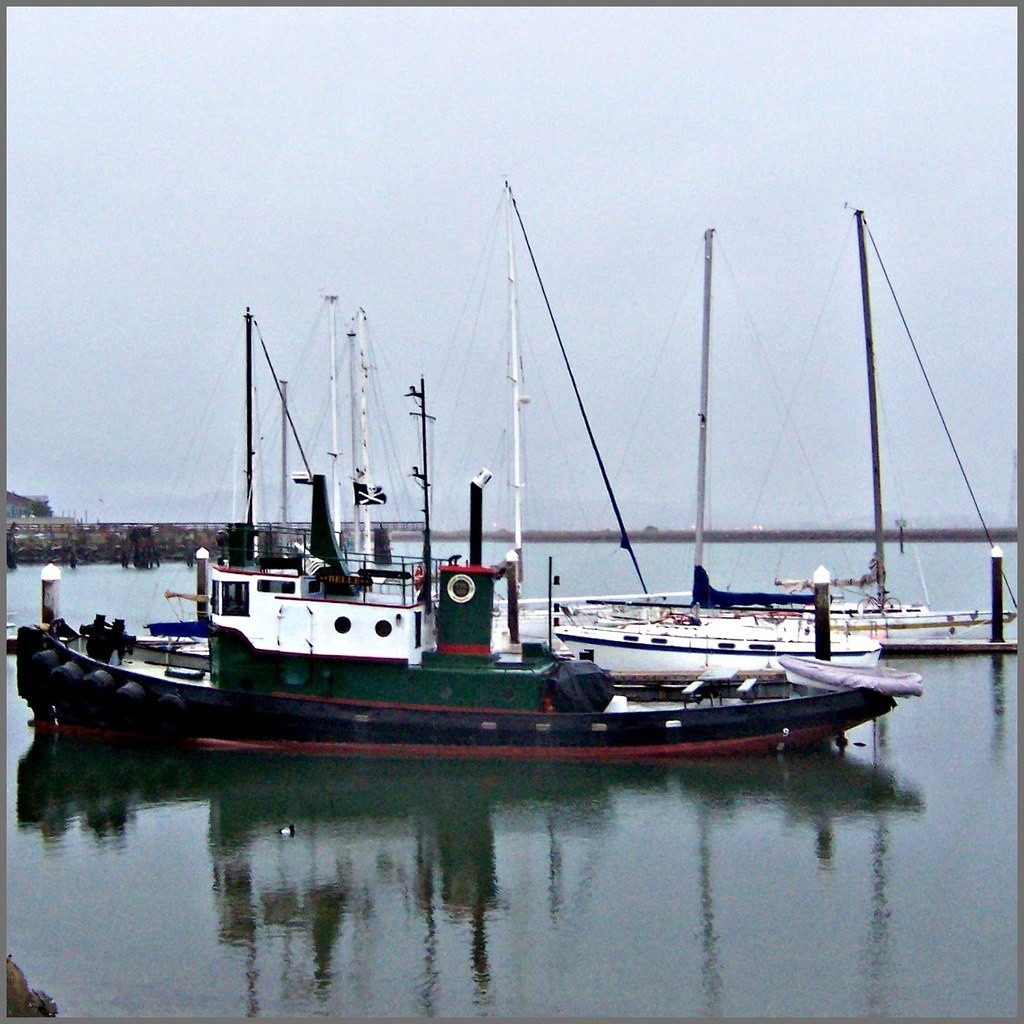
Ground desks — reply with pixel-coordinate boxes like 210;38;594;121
684;666;756;709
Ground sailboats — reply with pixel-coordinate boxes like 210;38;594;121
142;172;1019;675
13;290;932;765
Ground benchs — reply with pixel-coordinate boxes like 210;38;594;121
736;679;757;692
682;681;704;694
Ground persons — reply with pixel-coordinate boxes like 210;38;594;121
6;521;20;570
124;546;195;570
69;551;78;569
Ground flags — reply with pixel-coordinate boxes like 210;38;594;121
353;481;387;506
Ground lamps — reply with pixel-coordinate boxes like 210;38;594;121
813;565;831;583
196;547;210;559
991;544;1003;558
505;549;519;562
41;563;60;580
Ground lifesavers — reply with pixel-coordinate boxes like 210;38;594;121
447;574;476;603
